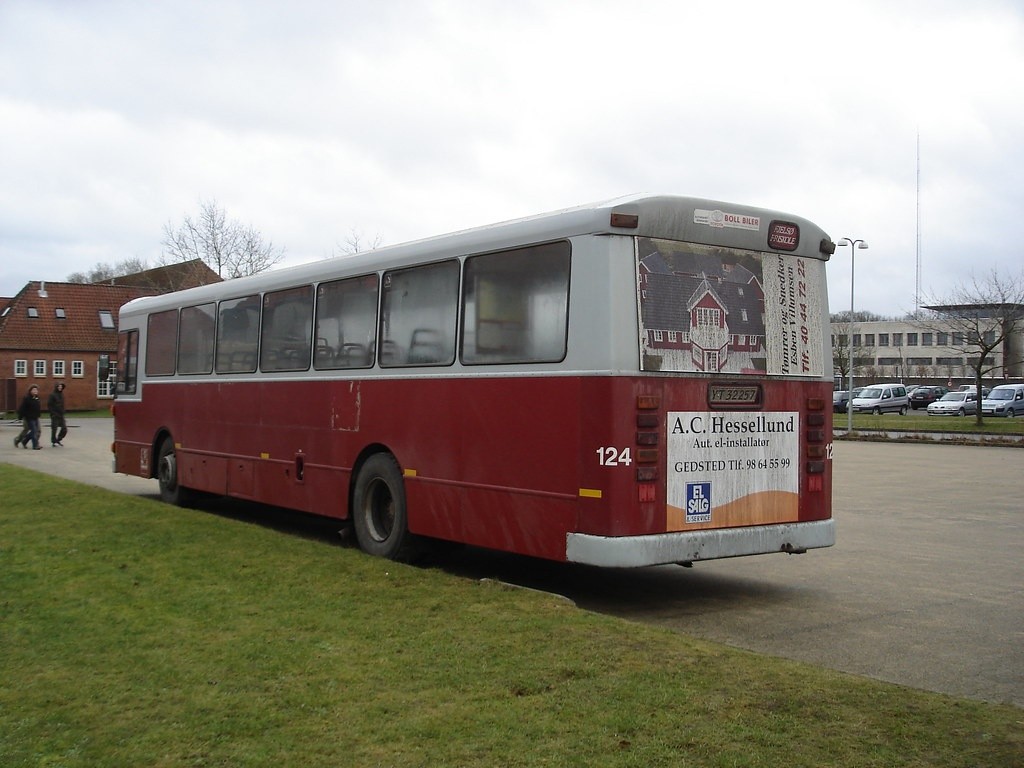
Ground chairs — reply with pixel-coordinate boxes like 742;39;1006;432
204;328;447;371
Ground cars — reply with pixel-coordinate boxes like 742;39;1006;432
926;392;980;418
832;389;860;414
845;383;910;415
981;383;1024;418
851;383;991;411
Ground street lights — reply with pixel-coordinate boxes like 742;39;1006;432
837;237;868;439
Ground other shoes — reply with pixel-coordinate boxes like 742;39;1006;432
32;447;41;450
56;440;64;446
21;439;28;449
52;443;57;447
13;437;20;448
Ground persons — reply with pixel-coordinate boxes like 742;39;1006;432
14;384;43;450
47;383;67;446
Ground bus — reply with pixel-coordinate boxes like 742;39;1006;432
96;195;838;575
